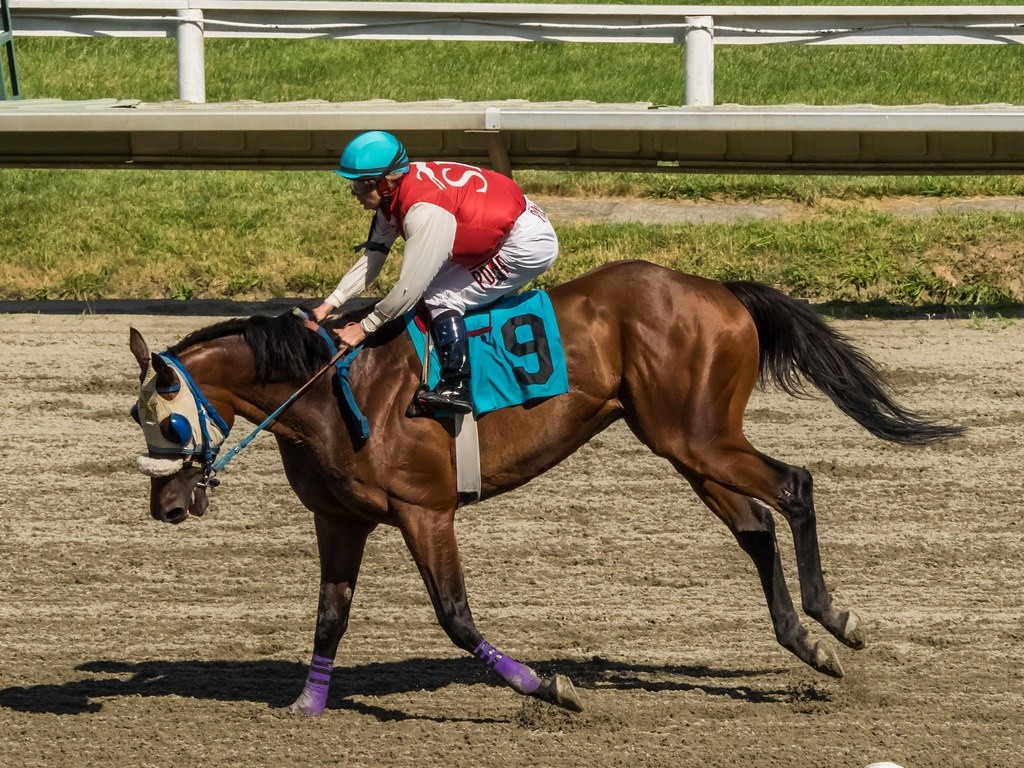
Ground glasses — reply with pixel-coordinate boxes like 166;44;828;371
350;179;377;194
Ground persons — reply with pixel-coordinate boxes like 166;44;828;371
311;131;558;415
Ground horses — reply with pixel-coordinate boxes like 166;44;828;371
131;258;969;722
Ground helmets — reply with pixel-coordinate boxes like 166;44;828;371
333;130;410;179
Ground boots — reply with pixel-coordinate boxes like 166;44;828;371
418;310;472;413
